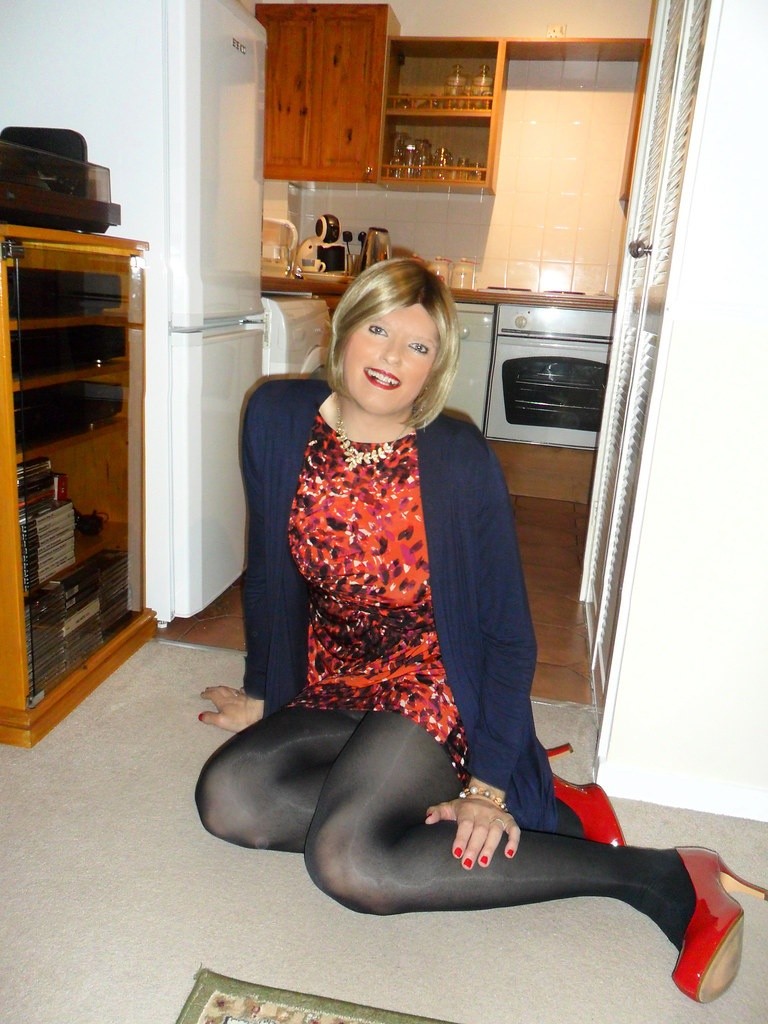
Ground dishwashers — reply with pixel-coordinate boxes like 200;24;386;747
441;303;496;431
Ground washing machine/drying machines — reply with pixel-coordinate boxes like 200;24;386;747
261;293;333;375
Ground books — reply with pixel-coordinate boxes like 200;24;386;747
16;458;130;698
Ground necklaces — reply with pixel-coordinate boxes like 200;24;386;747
336;406;409;472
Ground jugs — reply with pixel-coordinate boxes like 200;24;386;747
261;217;299;280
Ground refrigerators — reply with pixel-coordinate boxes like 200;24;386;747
0;0;268;626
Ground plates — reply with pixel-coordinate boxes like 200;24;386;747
303;273;344;281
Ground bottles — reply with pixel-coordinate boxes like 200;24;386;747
387;131;483;179
471;64;492;108
442;63;467;106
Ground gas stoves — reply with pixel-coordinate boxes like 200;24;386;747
483;285;615;302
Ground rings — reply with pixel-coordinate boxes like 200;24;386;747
494;818;504;826
234;692;240;696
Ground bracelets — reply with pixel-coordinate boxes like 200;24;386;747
459;786;509;812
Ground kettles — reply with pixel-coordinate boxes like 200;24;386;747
353;226;392;279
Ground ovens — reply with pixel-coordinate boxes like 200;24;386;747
484;302;615;451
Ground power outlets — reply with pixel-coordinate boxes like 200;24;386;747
342;225;365;245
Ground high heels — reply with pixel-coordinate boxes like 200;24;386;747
545;744;626;850
670;843;768;1004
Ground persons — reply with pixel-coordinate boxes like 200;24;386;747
195;258;768;1004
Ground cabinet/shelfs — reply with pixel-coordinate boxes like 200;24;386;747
253;5;508;192
0;224;158;748
